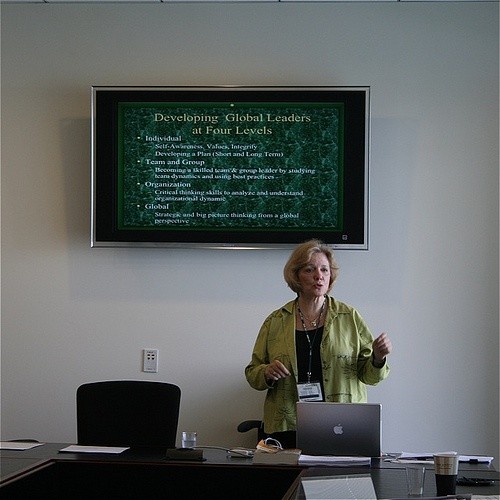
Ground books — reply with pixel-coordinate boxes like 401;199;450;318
253;440;371;465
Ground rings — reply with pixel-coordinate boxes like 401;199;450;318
272;370;276;375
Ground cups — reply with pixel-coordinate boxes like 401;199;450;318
181;431;197;449
433;453;459;500
405;466;427;496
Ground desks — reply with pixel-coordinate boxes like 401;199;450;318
0;441;500;500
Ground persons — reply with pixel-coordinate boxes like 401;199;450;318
245;241;392;450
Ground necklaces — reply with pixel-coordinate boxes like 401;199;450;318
300;298;327;326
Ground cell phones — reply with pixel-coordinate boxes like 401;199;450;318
229;450;254;457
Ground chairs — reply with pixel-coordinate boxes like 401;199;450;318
236;419;297;451
76;379;181;447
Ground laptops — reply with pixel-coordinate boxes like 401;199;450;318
296;402;382;457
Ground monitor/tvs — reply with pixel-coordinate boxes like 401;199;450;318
90;85;370;251
